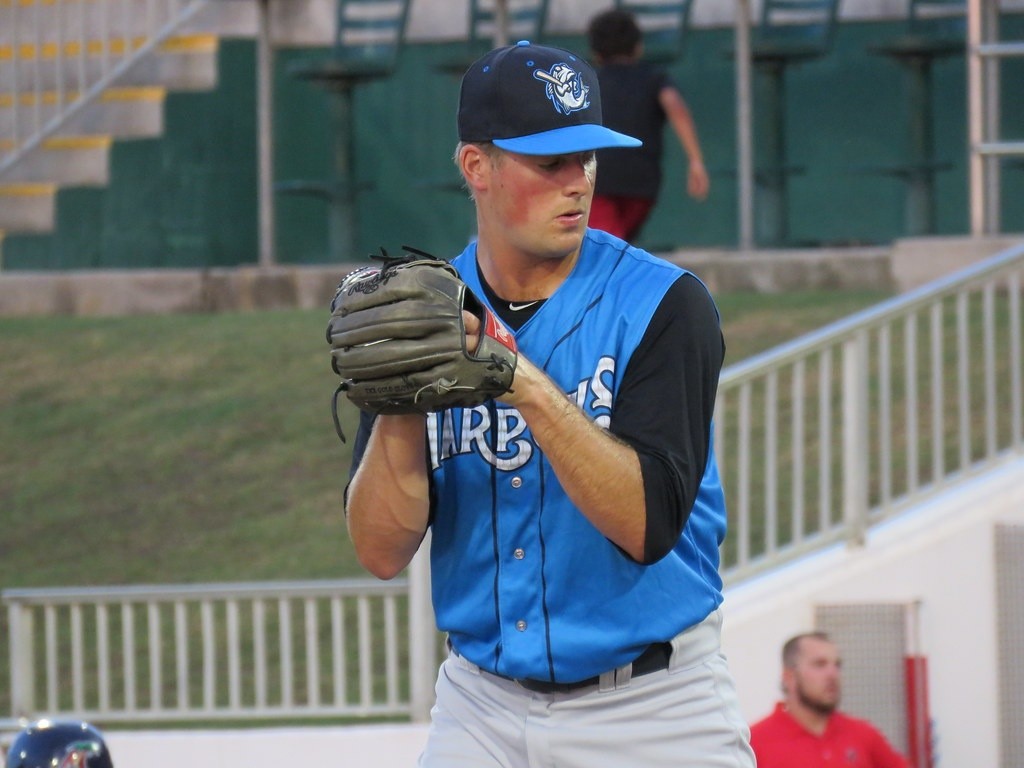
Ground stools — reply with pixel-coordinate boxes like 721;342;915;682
724;0;840;247
855;0;965;235
277;0;411;260
433;0;551;195
619;0;691;253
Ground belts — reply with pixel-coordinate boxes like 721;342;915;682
445;638;672;692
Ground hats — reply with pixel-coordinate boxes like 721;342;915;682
458;39;644;157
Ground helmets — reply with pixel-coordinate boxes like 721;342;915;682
4;722;113;768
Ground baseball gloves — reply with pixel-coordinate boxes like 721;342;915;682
326;259;519;417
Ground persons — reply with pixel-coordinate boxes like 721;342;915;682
749;633;912;768
326;41;758;768
587;10;711;245
